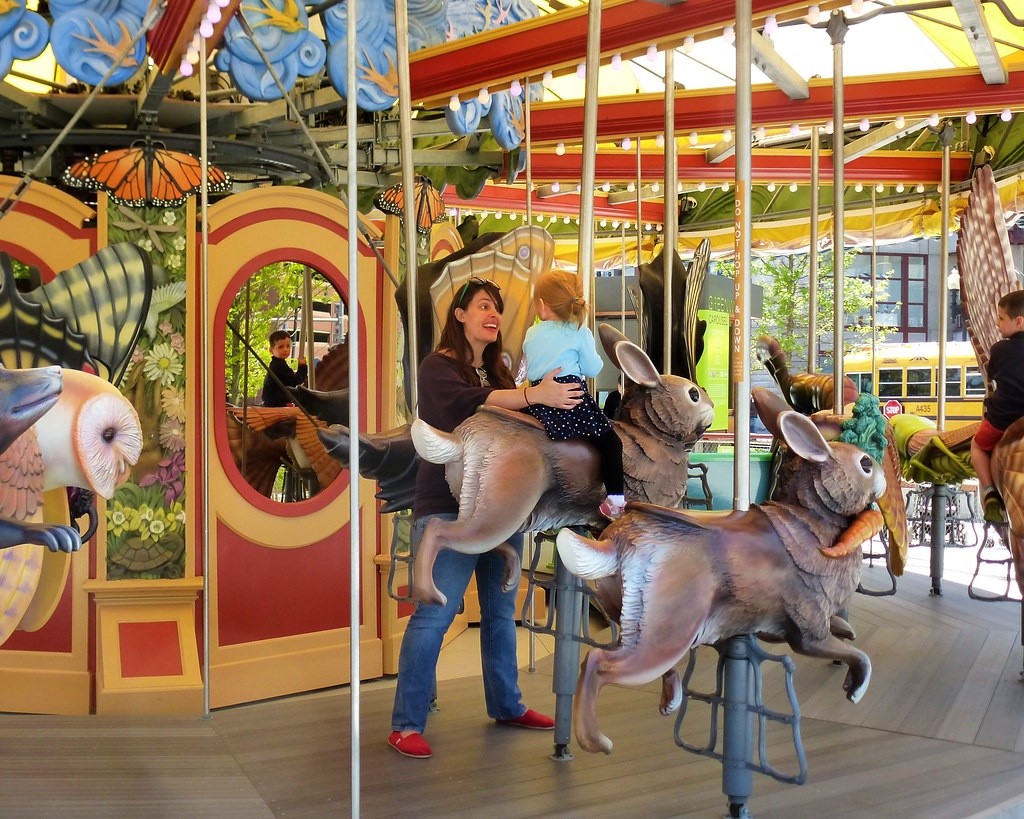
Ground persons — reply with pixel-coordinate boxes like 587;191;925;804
387;276;585;760
261;331;307;471
970;290;1024;524
523;270;627;523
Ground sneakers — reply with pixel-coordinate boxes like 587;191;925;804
598;498;628;521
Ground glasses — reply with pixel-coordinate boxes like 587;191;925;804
458;277;501;307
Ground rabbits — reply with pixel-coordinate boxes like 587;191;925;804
407;316;718;612
553;386;888;758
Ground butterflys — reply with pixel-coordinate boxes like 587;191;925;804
59;132;236;211
372;171;449;234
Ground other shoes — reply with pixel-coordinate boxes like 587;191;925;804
496;706;555;730
984;493;1004;523
388;730;433;758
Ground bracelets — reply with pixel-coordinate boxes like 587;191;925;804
524;387;531;406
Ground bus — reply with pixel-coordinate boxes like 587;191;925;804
844;340;986;431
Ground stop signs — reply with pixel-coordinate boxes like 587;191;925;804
885;400;903;420
